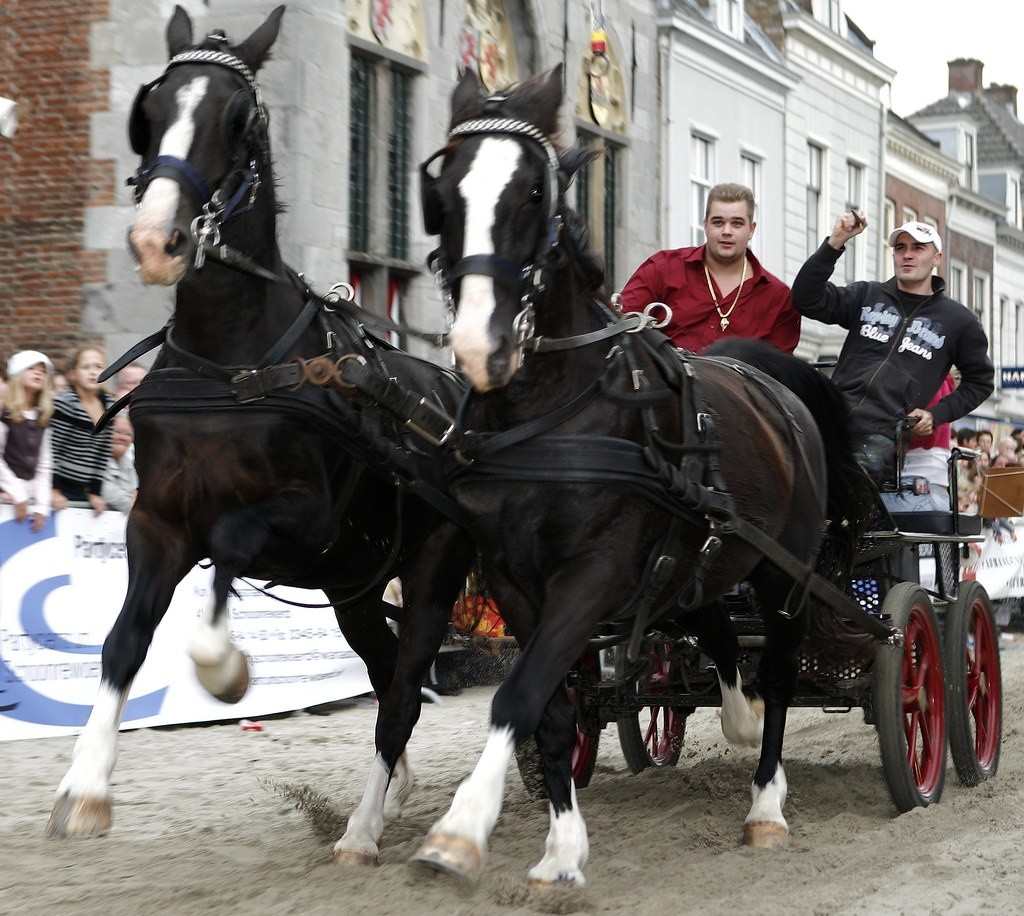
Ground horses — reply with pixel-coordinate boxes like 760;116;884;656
44;4;827;897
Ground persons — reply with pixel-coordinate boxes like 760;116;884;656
617;184;801;359
949;427;1024;646
790;210;995;595
0;347;148;532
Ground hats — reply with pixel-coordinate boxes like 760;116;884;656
887;220;943;256
7;350;54;377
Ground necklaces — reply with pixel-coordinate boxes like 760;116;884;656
704;254;747;332
